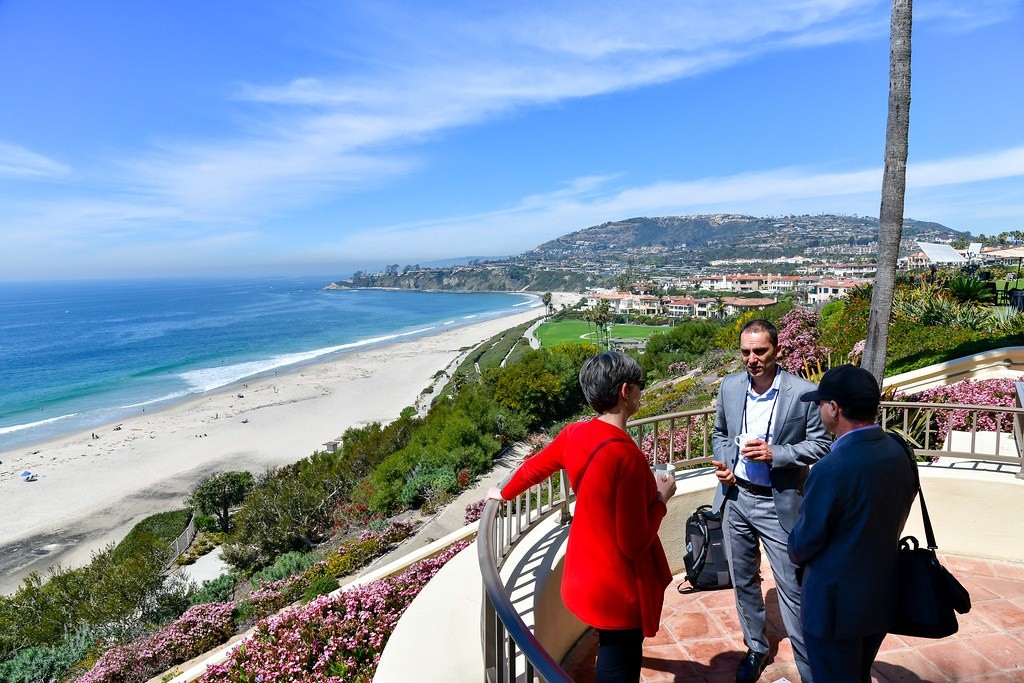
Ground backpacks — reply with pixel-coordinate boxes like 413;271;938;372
677;505;733;594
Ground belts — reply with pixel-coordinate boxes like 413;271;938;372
734;474;773;496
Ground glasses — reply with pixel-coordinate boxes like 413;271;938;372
815;400;831;405
620;378;645;391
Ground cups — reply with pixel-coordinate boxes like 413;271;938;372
734;433;759;454
653;464;675;496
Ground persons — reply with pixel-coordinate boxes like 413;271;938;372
484;352;677;683
787;363;919;683
712;319;835;683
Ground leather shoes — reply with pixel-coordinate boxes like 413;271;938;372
736;648;768;683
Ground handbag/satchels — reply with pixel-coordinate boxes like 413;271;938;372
887;536;971;638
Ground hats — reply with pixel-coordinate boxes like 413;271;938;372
800;365;879;407
